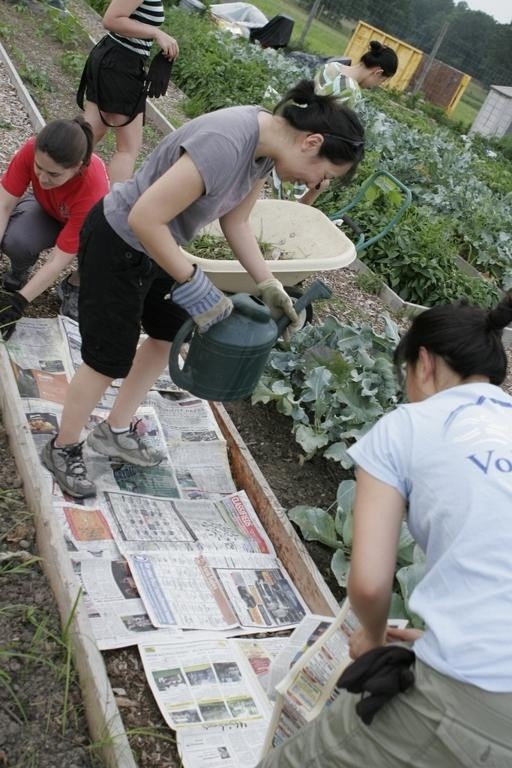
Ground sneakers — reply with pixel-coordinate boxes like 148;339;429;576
41;433;97;498
3;253;39;293
88;419;163;467
58;273;80;323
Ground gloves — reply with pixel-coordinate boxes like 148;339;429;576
165;264;233;334
146;49;173;98
258;279;298;342
337;646;415;725
0;291;28;341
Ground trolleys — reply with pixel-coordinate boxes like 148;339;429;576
177;166;413;352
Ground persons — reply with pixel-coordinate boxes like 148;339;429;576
273;41;397;203
0;114;111;340
39;81;363;500
75;1;182;191
254;299;511;768
